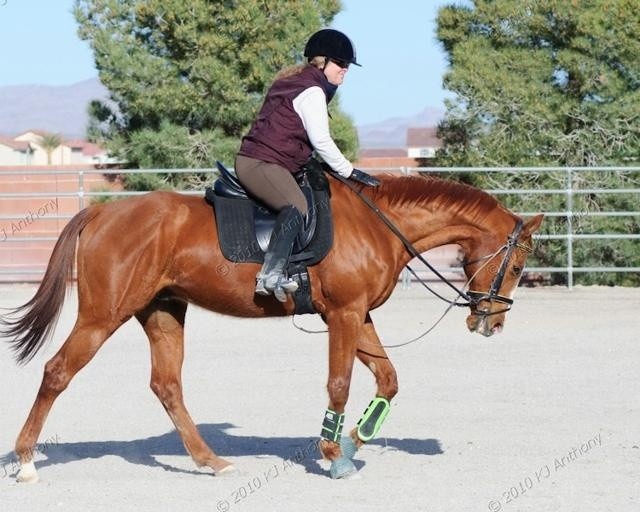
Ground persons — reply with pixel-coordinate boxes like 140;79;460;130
234;30;382;296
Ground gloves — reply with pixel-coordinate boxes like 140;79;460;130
349;168;380;188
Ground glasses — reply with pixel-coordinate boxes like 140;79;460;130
331;59;350;67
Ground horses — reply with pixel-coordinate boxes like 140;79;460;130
0;173;544;485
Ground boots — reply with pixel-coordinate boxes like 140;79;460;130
255;205;299;297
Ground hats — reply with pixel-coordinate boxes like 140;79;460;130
303;28;362;68
